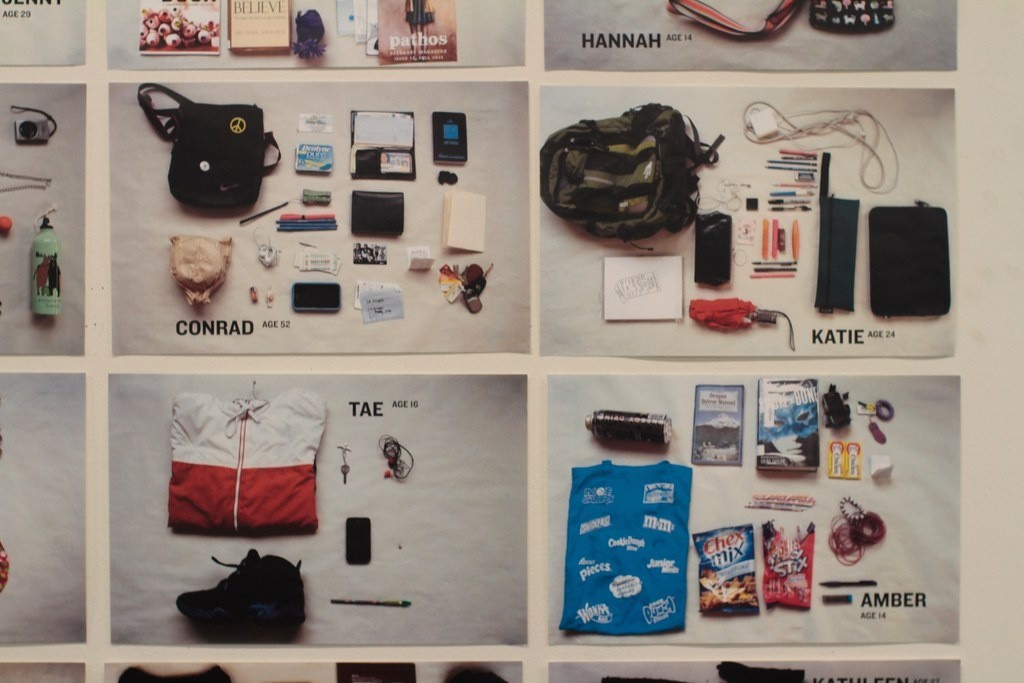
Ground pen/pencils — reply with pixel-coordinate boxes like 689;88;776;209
819;580;878;588
750;260;798;279
769;183;817;196
764;150;818;171
329;599;411;607
768;206;812;211
275;214;338;232
238;201;289;225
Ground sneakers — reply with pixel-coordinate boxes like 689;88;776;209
176;548;307;637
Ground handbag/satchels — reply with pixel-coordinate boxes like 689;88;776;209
809;0;895;34
137;82;281;211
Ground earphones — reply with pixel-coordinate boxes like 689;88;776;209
384;470;392;477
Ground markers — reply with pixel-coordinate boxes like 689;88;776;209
762;218;778;258
766;198;814;204
793;219;799;259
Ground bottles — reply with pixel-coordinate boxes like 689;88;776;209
586;410;671;446
29;216;63;315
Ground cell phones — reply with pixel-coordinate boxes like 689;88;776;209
292;282;341;310
345;517;371;565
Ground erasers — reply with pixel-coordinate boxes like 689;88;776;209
794;173;815;181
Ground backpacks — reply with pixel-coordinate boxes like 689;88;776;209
538;102;726;251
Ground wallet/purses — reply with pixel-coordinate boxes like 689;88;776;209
351;190;405;237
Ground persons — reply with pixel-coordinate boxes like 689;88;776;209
382;154;390;167
766;381;818;466
354;243;385;264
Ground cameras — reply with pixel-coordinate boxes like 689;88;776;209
15;118;49;141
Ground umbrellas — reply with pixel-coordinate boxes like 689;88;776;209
689;298;795;352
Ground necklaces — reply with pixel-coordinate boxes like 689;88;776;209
0;172;51;192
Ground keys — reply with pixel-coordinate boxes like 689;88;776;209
341;465;350;485
462;263;493;314
823;392;850;428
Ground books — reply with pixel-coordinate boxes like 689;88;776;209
227;0;291;52
140;0;220;55
757;378;820;472
442;191;485;252
336;0;457;64
